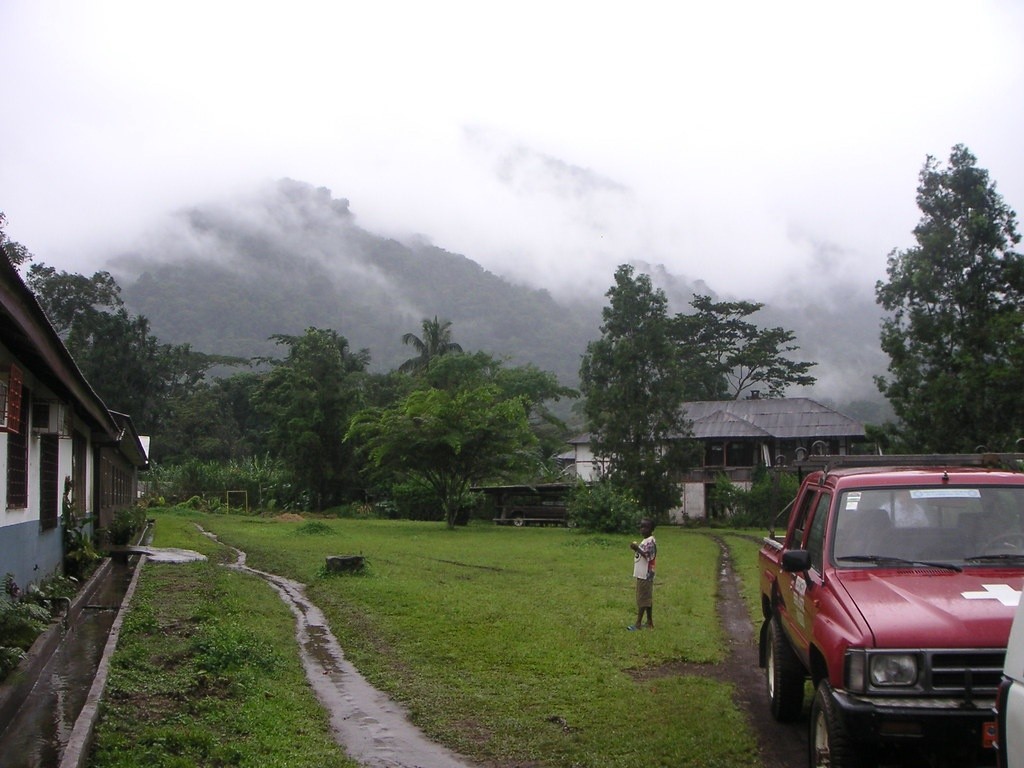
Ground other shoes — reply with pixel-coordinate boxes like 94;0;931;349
627;625;637;630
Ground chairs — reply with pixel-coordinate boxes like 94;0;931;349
844;511;909;559
959;510;1009;557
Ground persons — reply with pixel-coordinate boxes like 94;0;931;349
881;491;929;527
627;518;657;630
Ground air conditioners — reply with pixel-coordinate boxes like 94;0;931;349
31;401;65;435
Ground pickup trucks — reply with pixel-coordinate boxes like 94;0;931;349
758;465;1024;768
